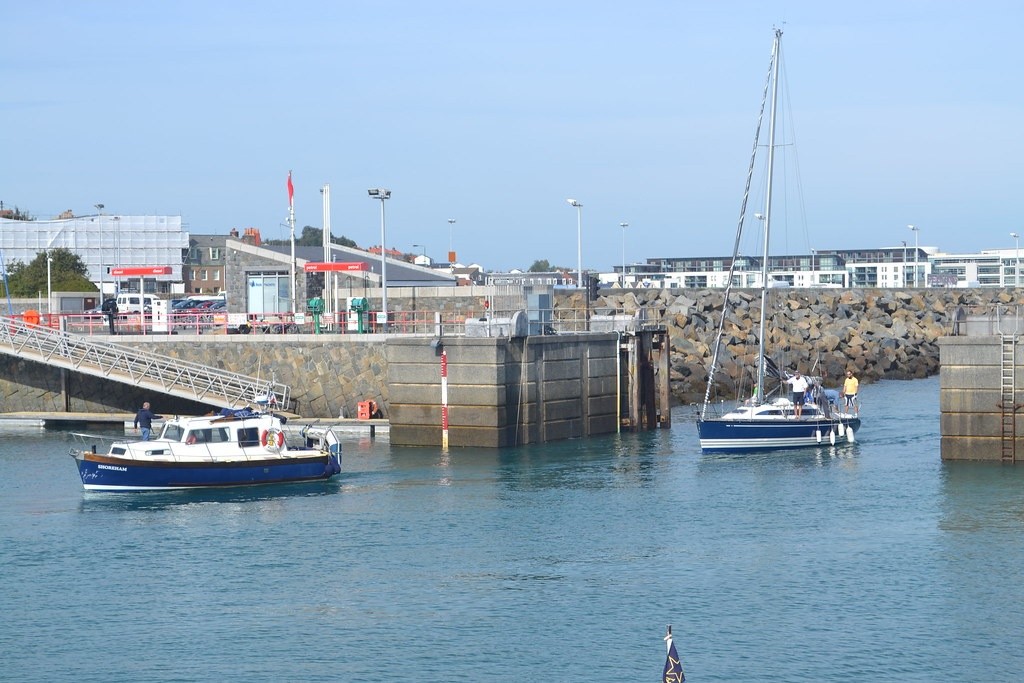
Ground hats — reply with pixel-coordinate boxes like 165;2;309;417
794;370;800;374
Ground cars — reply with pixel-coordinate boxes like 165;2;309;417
84;304;110;319
172;300;226;309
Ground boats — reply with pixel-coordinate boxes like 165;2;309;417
67;357;342;490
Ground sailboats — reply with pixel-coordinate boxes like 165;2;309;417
696;27;862;452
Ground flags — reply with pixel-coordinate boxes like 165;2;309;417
287;173;293;205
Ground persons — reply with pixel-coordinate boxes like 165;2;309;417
134;402;165;441
782;371;808;418
753;382;758;397
811;381;824;411
843;370;858;418
186;430;197;445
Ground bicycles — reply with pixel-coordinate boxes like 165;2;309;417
272;314;299;333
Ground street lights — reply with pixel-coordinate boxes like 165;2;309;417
413;244;425;266
907;225;922;287
811;248;815;285
567;199;583;287
448;219;456;249
47;257;54;315
1009;233;1020;287
621;222;629;288
93;204;105;304
368;188;391;332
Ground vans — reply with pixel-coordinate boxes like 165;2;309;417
177;300;205;309
116;293;161;314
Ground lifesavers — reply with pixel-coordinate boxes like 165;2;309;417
365;399;378;415
261;427;284;452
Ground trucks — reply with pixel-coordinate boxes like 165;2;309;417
181;295;224;300
